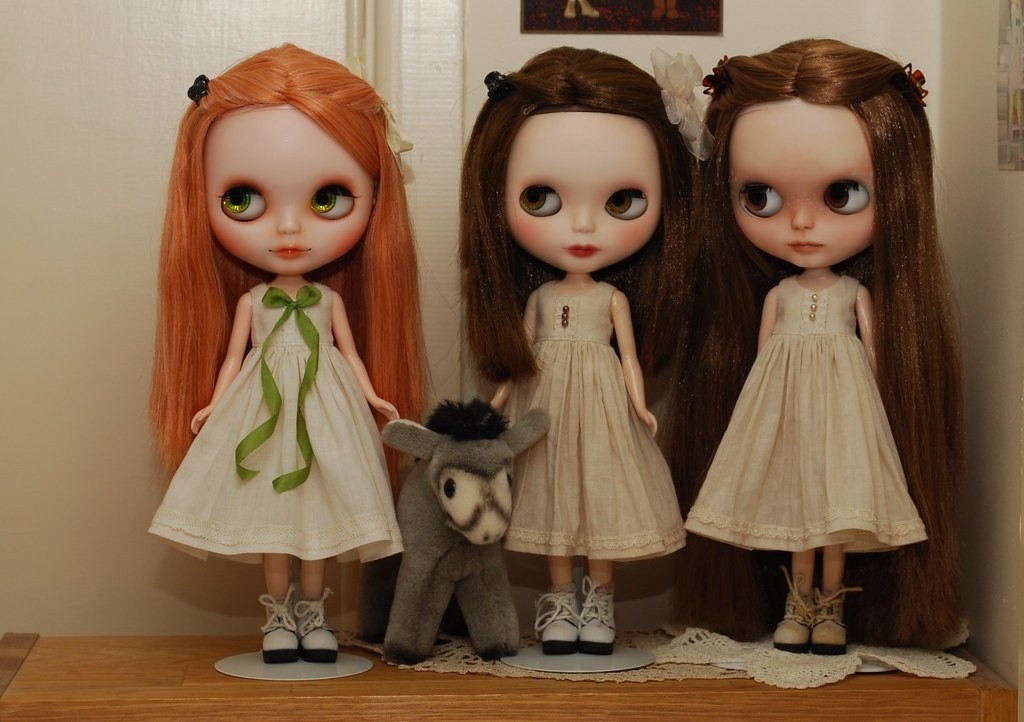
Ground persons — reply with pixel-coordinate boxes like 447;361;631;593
663;36;961;653
457;45;715;653
148;45;431;663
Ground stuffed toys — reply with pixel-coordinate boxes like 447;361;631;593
357;399;551;661
